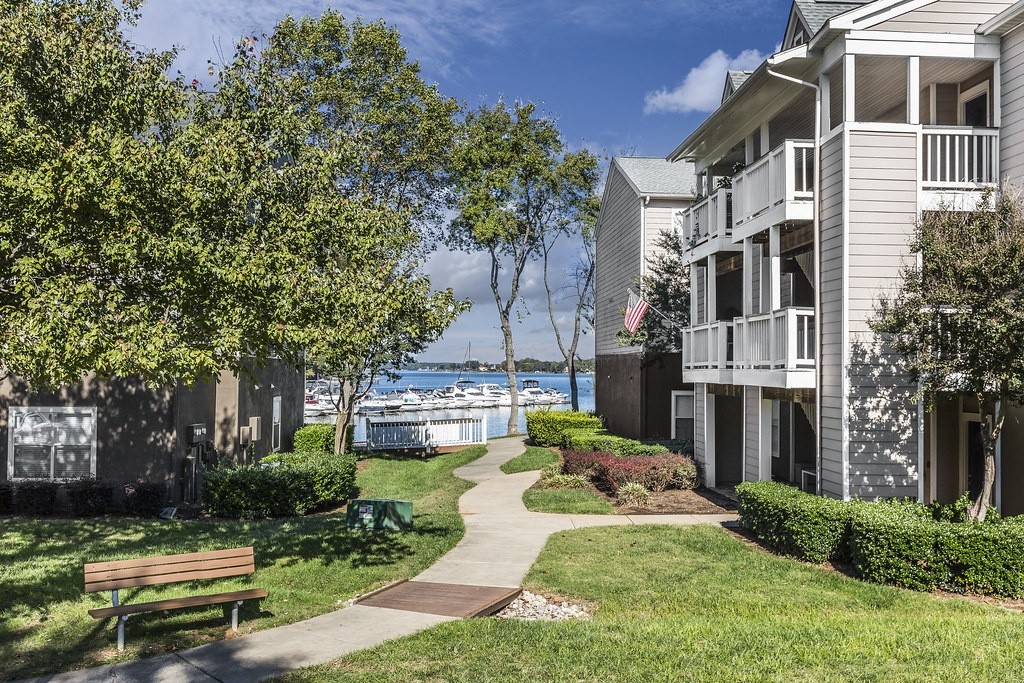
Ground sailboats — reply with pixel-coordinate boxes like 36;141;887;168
457;340;478;384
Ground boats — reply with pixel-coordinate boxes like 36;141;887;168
305;377;571;418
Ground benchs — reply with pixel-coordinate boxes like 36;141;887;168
365;417;436;454
84;547;269;652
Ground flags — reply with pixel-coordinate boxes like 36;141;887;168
624;290;650;334
